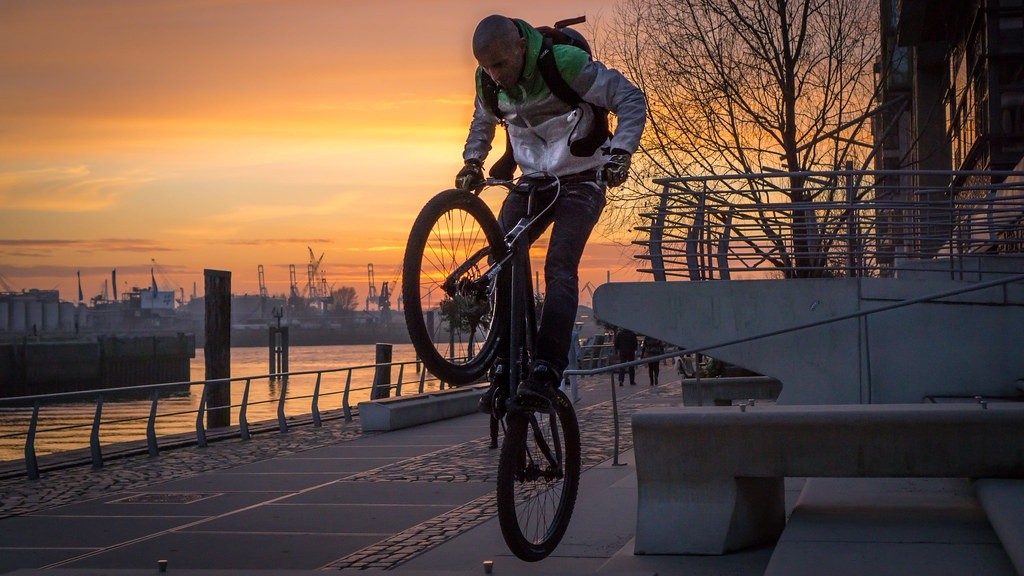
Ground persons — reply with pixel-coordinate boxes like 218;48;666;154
559;331;582;402
640;337;665;386
487;366;498;449
454;15;646;414
614;328;638;385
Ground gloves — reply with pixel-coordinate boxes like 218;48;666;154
596;151;632;189
455;160;485;197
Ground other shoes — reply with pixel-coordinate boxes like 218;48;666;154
478;362;563;416
650;381;659;386
619;382;637;386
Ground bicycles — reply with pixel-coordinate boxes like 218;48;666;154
401;169;598;562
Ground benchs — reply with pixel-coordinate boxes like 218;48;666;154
681;376;780;408
632;402;1024;556
357;382;491;432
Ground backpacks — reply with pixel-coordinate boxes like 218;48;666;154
481;26;597;120
